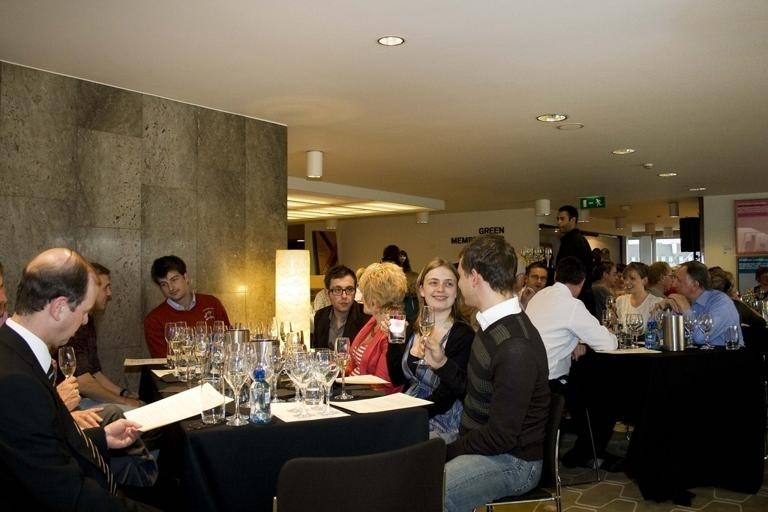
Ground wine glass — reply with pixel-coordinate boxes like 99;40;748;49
602;295;714;351
519;247;553;268
165;319;355;426
414;305;436;366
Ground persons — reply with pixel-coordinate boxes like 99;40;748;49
308;205;768;512
147;255;231;359
0;248;160;511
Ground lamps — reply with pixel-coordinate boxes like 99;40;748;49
533;198;551;217
415;211;429;224
304;148;325;180
575;201;680;239
273;248;312;352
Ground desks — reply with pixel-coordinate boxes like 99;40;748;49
570;339;765;505
134;363;431;512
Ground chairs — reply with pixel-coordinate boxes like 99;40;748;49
473;390;564;511
267;434;449;512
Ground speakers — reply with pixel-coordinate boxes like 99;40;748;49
679;217;700;251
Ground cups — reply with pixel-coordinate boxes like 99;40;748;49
57;344;78;379
725;325;739;349
735;288;768;328
389;310;407;344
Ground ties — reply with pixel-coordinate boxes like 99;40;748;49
46;358;118;498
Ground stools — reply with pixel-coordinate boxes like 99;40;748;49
564;396;603;485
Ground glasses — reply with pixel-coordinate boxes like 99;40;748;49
329;286;355;296
